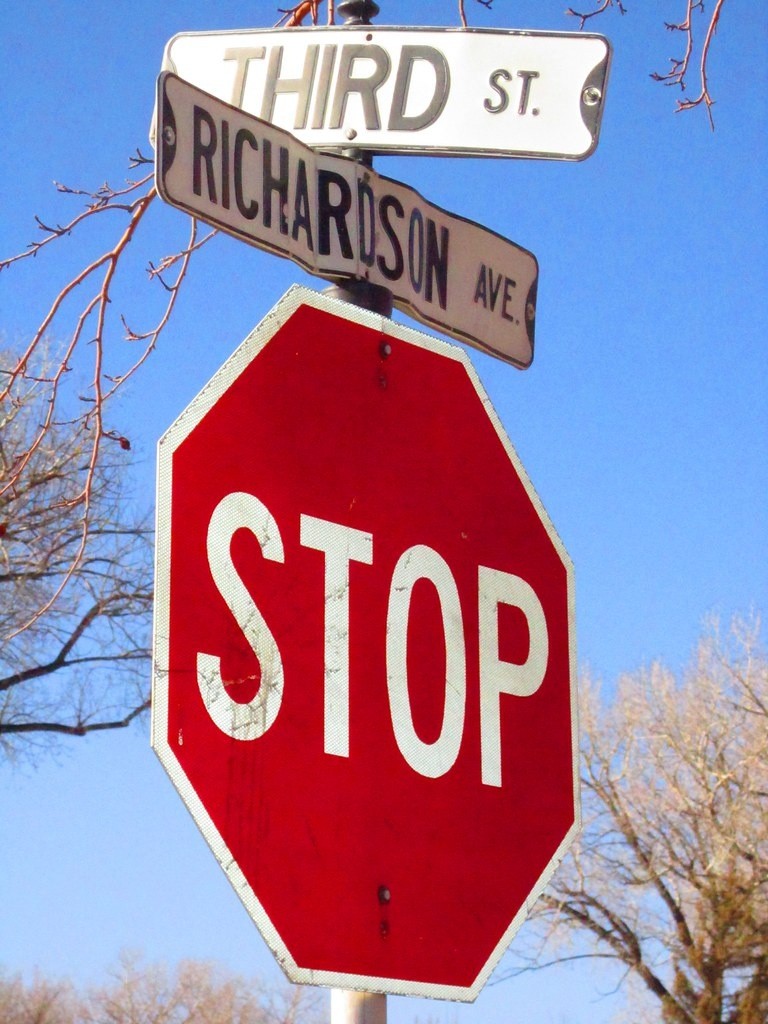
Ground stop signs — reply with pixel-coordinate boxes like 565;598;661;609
149;285;581;1002
